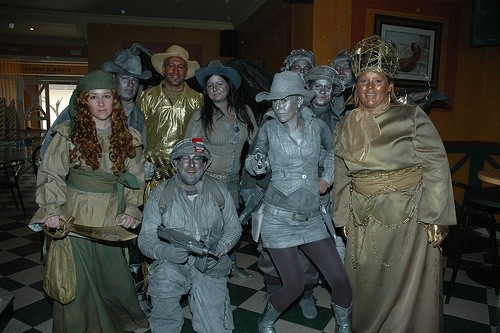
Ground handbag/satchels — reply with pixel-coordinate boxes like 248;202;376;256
42;230;76;305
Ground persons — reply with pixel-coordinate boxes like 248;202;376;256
184;60;259;279
42;41;354;333
28;70;146;333
329;36;456;333
243;71;353;333
137;138;243;332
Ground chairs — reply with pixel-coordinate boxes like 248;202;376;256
32;145;42;178
0;159;26;218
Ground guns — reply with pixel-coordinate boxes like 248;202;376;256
254;147;262;169
157;223;220;258
239;190;264;222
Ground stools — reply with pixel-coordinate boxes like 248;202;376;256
446;189;500;306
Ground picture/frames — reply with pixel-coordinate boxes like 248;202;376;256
121;39;204;68
373;14;442;91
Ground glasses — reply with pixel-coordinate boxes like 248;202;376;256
179;156;204;164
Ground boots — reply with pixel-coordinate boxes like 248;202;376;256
331;298;354;333
256;292;282;333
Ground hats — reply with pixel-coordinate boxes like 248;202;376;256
305;65;345;96
151;44;200;80
349;35;400;79
169;136;213;171
330;48;351;70
255;71;316;102
103;49;153;80
194;60;242;90
280;48;316;72
78;70;117;91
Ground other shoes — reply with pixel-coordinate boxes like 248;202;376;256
230;266;254;278
298;295;318;319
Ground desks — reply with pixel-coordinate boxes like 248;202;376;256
476;170;500;187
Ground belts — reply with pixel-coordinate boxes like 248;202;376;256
263;205;322;223
